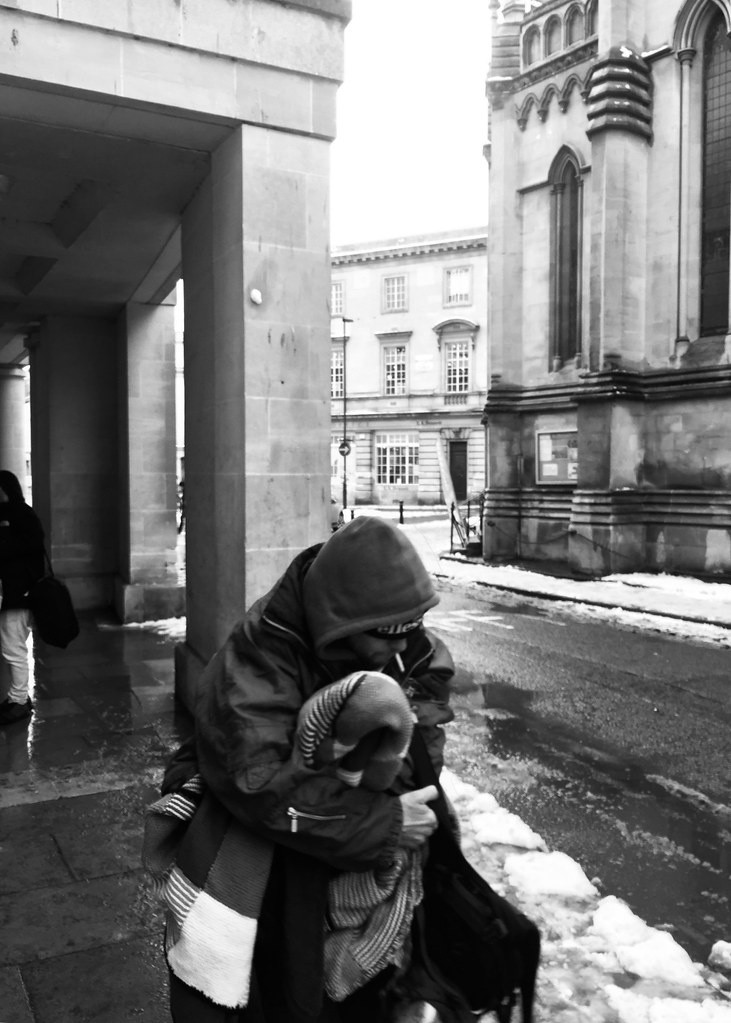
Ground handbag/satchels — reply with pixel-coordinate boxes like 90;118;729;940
34;579;79;648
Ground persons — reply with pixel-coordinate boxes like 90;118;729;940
0;470;44;733
137;514;459;1022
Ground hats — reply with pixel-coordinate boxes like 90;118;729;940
364;612;422;638
